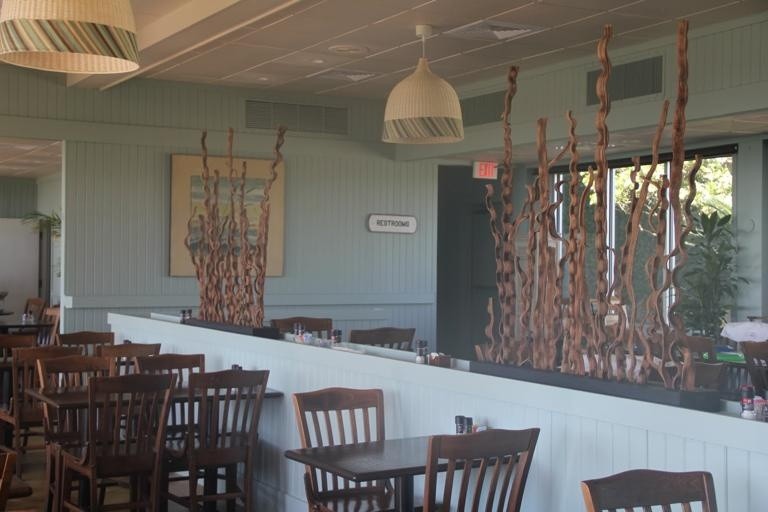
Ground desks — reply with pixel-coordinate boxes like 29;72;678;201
283;435;520;511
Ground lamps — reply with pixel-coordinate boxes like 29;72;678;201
380;22;465;149
1;0;143;78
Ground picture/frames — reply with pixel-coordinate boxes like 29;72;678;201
166;150;287;280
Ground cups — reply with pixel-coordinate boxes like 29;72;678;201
288;331;327;344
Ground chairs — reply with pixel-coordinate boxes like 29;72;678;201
0;296;286;512
290;386;447;512
423;428;540;511
578;469;720;511
268;317;415;352
557;297;767;402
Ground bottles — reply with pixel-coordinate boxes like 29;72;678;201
21;310;33;321
455;415;473;435
294;321;305;337
416;356;425;364
415;339;428;357
330;328;342;342
231;364;242;370
122;339;131;345
760;386;768;417
179;309;192;323
740;384;754;412
